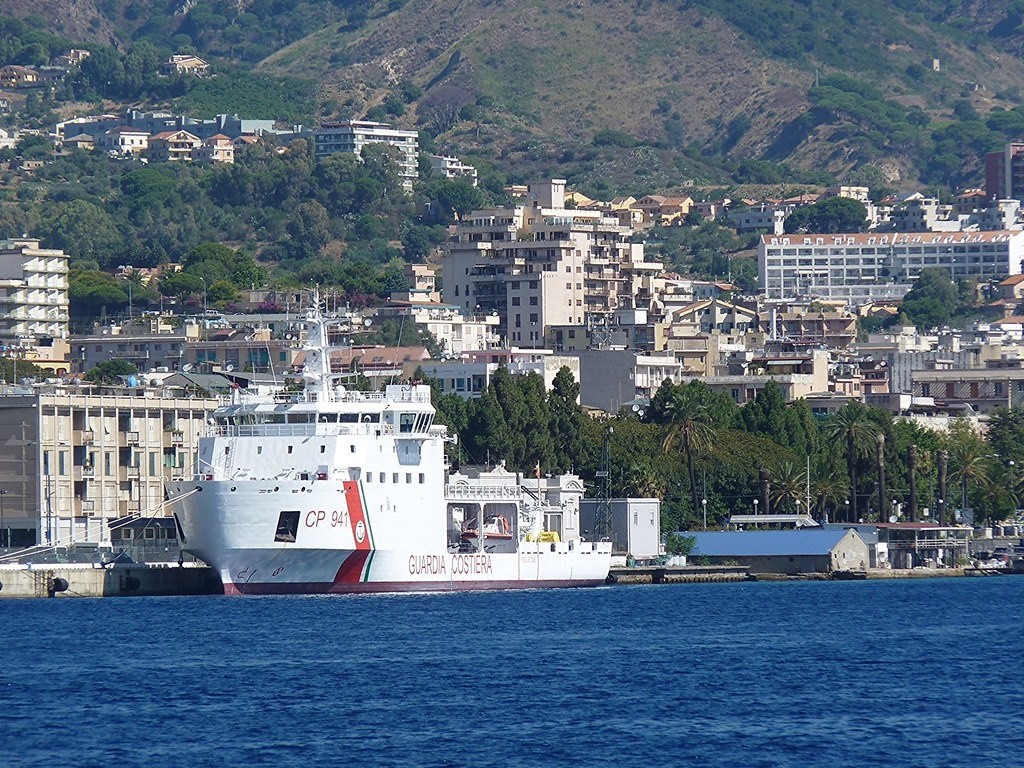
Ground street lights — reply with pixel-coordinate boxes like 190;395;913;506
844;499;850;523
938;498;943;527
754;499;759;528
702;499;707;531
795;500;800;517
891;498;898;514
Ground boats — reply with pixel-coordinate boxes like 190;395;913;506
161;282;616;593
833;566;868;580
